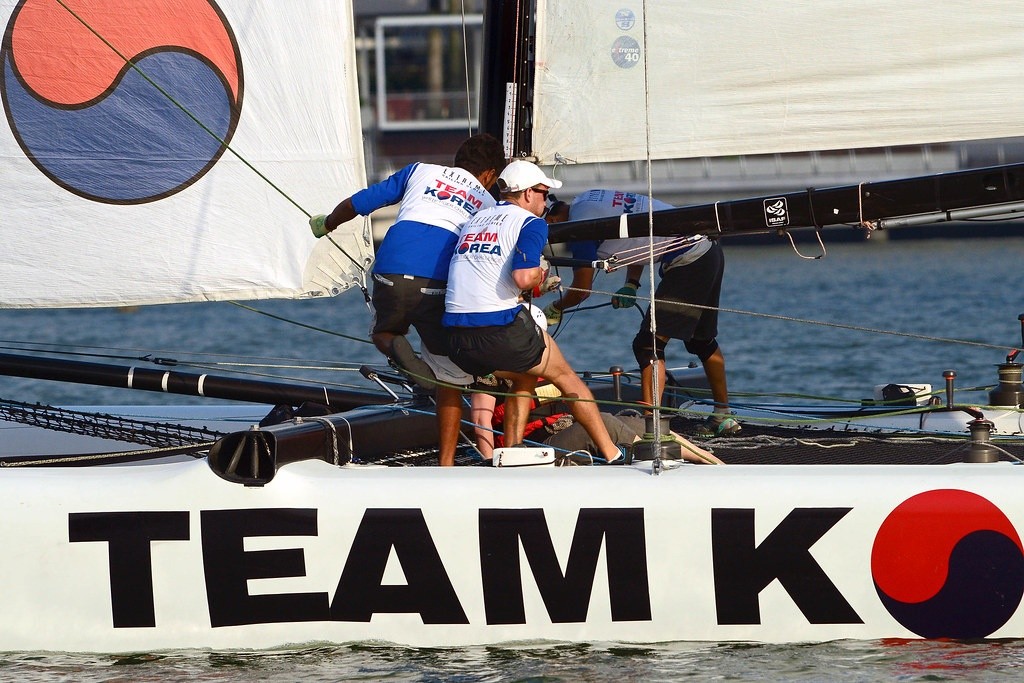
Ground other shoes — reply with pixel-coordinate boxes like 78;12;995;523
603;442;635;465
391;334;436;390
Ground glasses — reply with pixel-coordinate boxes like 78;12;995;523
524;187;549;201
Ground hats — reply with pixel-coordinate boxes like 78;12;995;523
541;191;560;219
497;160;562;192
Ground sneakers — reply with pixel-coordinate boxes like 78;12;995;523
705;411;742;437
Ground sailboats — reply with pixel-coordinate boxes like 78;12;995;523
0;0;1024;655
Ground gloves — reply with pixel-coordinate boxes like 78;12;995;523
533;276;563;297
612;283;637;309
309;214;331;238
542;301;563;326
539;255;552;286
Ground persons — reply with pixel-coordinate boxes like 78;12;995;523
419;336;504;465
545;190;742;436
442;159;634;466
309;132;504;466
